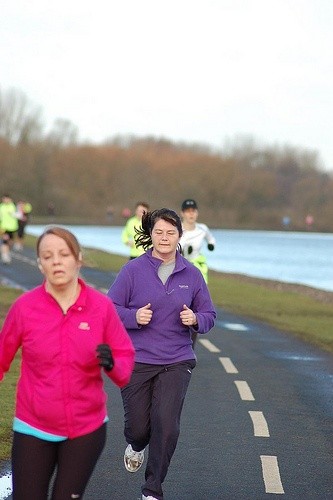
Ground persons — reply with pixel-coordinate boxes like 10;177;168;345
282;214;290;230
0;193;33;264
106;198;217;500
306;213;313;231
0;227;136;500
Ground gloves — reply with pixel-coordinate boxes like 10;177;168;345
208;244;214;251
95;344;113;372
187;246;192;255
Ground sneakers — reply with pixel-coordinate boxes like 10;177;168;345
140;493;158;500
123;444;145;473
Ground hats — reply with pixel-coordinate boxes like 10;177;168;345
181;199;197;211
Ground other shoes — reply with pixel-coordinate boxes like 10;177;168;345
1;255;12;264
13;244;23;251
